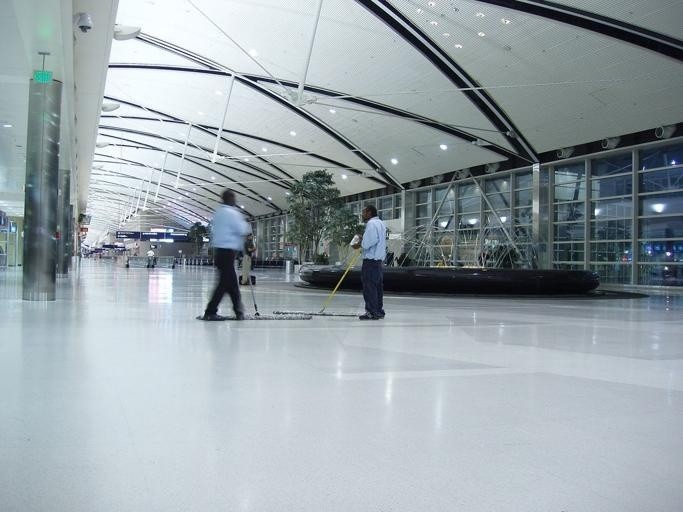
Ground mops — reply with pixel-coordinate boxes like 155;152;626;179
195;250;311;322
273;249;366;320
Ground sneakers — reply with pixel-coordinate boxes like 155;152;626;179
235;313;244;320
359;312;376;319
203;313;223;321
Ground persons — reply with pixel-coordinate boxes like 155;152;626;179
145;250;154;268
350;205;385;320
385;244;520;268
239;233;257;285
202;188;253;321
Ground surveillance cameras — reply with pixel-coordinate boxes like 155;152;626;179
77;13;93;32
